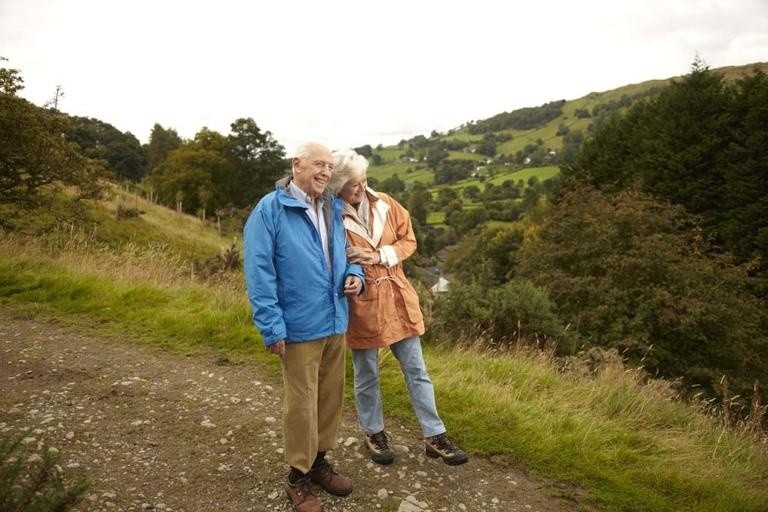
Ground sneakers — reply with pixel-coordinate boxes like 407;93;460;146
310;462;353;495
365;431;393;464
426;433;468;466
286;473;324;512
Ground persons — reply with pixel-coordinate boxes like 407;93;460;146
328;147;467;466
241;141;366;512
